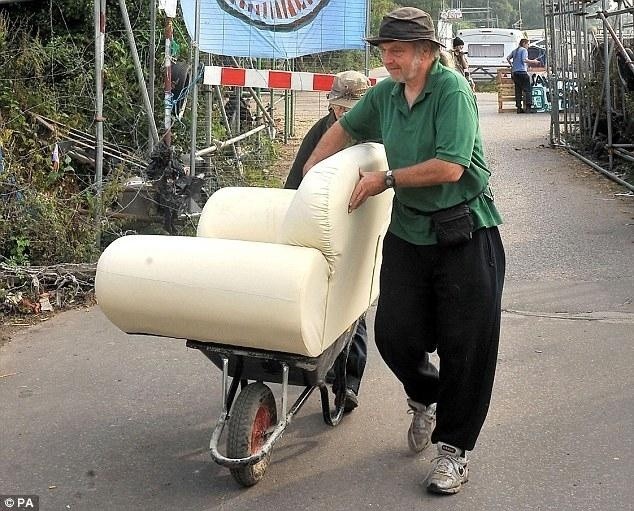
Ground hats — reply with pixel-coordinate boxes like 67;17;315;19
364;6;447;48
326;70;373;110
453;36;464;47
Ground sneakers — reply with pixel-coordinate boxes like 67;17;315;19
517;108;537;114
425;441;470;495
406;397;437;455
334;388;359;414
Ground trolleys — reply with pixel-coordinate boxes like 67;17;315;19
185;311;380;486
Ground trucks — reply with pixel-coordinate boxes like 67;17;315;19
457;29;527;84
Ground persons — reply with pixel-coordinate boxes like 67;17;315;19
449;37;469;77
283;71;371;411
303;5;506;496
507;39;540;113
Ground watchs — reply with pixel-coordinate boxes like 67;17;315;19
385;171;396;190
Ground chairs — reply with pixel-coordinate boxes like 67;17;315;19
497;68;526;113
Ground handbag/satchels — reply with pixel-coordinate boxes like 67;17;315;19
431;202;475;249
